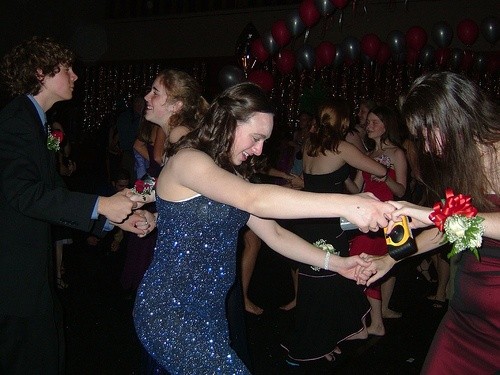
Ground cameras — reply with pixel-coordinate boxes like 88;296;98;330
383;215;418;261
340;217;359;230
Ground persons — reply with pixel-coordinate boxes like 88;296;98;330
238;101;462;340
49;120;71;289
125;69;211;238
87;87;166;257
355;72;500;375
280;103;389;361
133;82;397;375
0;33;147;375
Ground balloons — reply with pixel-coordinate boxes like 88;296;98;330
217;0;347;91
330;16;500;74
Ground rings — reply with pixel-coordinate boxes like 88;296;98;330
369;226;378;229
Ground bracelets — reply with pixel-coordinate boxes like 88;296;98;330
310;239;341;271
429;189;485;263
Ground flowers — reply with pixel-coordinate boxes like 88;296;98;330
438;214;486;262
374;155;394;169
310;239;338;272
129;176;158;201
46;124;64;152
